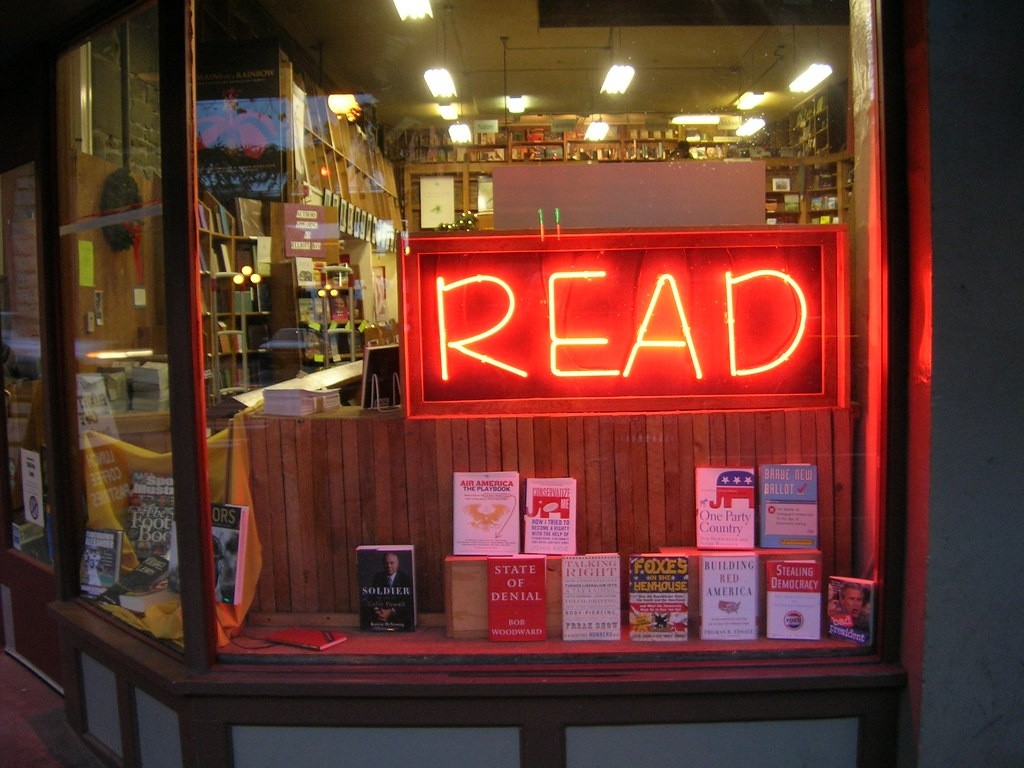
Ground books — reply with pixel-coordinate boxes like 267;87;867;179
13;335;822;652
828;576;873;643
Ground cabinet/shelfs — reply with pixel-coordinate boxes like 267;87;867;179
155;44;856;430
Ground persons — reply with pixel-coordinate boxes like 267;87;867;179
828;584;868;634
364;552;413;628
